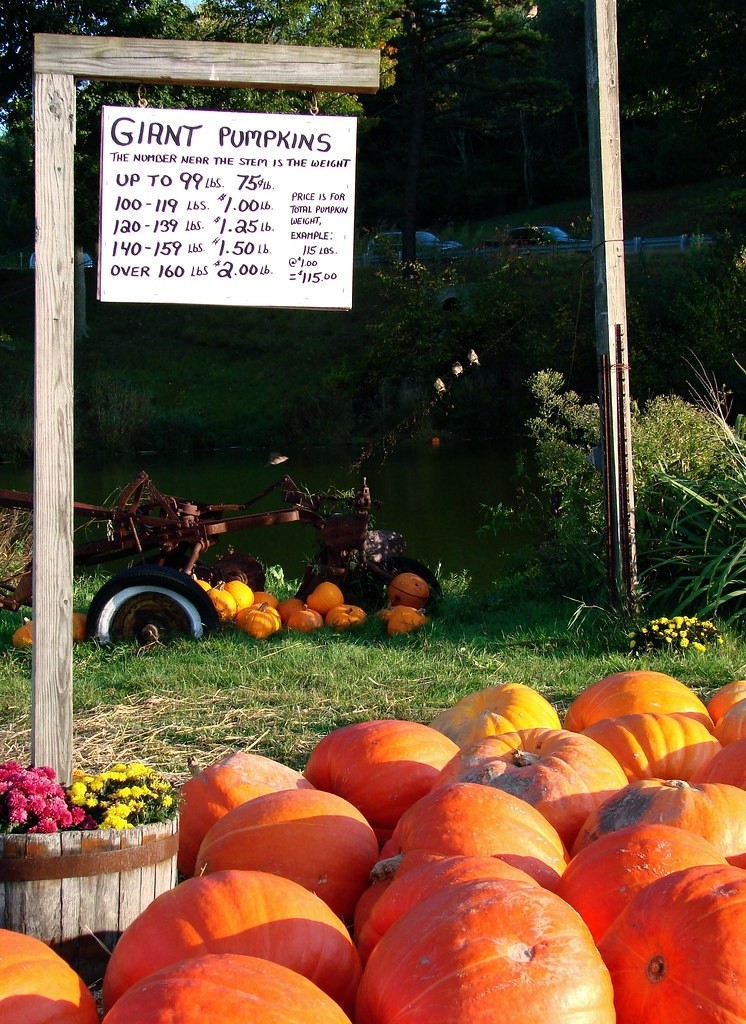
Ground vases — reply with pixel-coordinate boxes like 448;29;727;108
0;812;181;988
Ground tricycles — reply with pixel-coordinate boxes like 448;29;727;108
1;473;447;652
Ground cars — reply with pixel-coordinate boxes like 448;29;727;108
367;231;463;259
485;226;588;247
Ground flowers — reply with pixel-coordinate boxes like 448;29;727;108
67;758;182;835
0;754;96;836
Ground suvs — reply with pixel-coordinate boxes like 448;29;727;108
28;249;96;270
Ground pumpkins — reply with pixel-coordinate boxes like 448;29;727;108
0;671;746;1024
12;610;86;647
195;572;429;636
